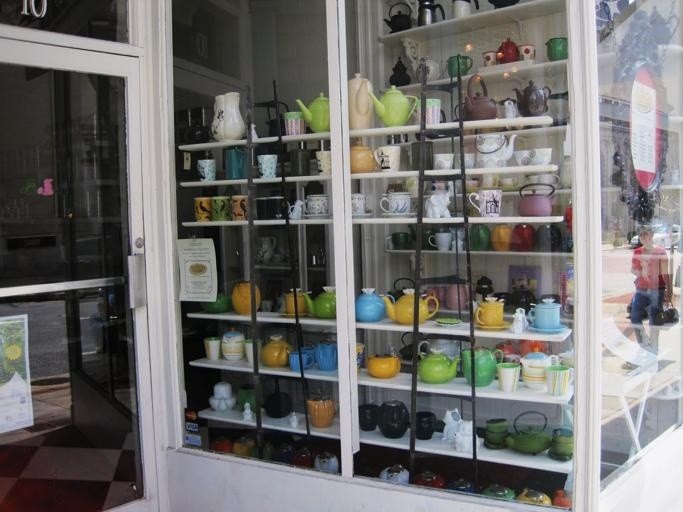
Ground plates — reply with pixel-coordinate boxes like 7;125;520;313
436;317;463;327
475;323;511;330
352;213;373;217
304;214;334;219
527;324;568;334
381;212;419;217
282;312;308;317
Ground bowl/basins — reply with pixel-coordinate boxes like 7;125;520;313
552;429;574;454
514;148;553;166
484;417;511;449
433;153;455;169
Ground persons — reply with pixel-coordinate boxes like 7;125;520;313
629;224;674;355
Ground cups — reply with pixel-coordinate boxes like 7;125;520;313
228;195;248;222
475;297;506;325
196;160;216;182
528;298;561;326
255;196;291;219
429;231;452;249
469;190;504;217
356;341;367;376
351;193;367;214
392;232;411;251
483;51;497;64
380;192;412;213
205;338;221;359
285;288;305;314
360;403;381;432
245;338;263;363
547;366;574;396
284;112;306;138
414;412;438;440
302;194;329;213
289;353;314;370
211;195;231;222
411;99;441;124
374;146;400;171
517;44;536;59
445;55;473;76
193;196;213;224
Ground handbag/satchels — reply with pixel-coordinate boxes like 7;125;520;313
655;301;679;326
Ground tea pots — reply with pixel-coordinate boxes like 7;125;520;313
379;464;576;511
380;289;439;325
419;354;461;383
416;0;446;24
233;280;261;316
387;277;415;302
355;288;396;322
211;431;342;475
315;152;331;175
520;352;558;390
469;223;562;254
381;3;416;32
496;37;520;61
264;383;295;418
513;80;553;117
503;411;554;454
518;183;555;216
369;84;418;126
511;284;538;313
418;338;461;364
261;335;294;366
497;363;521;392
475;134;517;168
199;294;232;313
416;55;446;84
349;73;374;128
257;154;278;179
465;75;496;122
367;354;400;380
307;398;339;428
462;347;504;387
451;0;480;21
311;340;340;371
303;286;336;319
208;382;238;415
296;92;330;133
397;331;431;374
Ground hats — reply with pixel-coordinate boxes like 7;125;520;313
638;225;654;238
